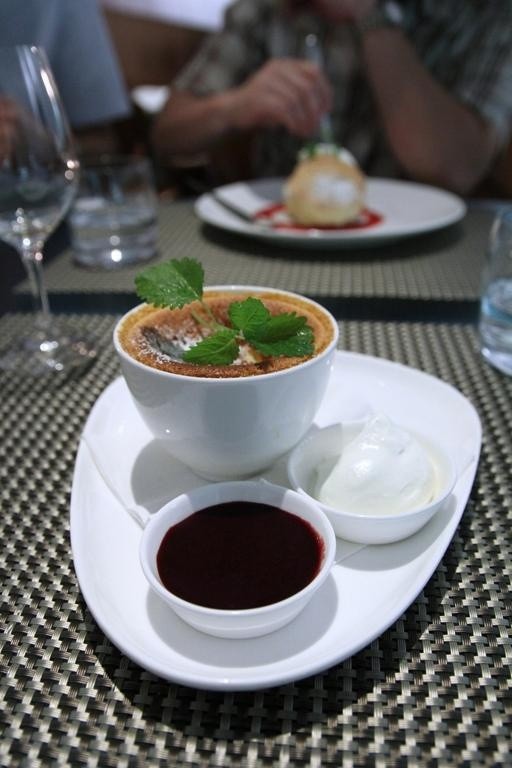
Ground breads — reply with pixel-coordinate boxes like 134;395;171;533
282;157;365;229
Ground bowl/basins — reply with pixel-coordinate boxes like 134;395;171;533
285;418;459;544
111;284;342;481
137;476;337;643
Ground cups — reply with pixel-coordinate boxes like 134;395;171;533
477;210;512;378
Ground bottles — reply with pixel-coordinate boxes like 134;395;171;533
34;1;162;272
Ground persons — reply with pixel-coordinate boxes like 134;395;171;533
0;0;133;287
148;0;512;200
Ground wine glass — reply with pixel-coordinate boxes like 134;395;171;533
0;42;99;375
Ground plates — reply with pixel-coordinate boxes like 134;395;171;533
66;346;485;695
191;172;470;252
